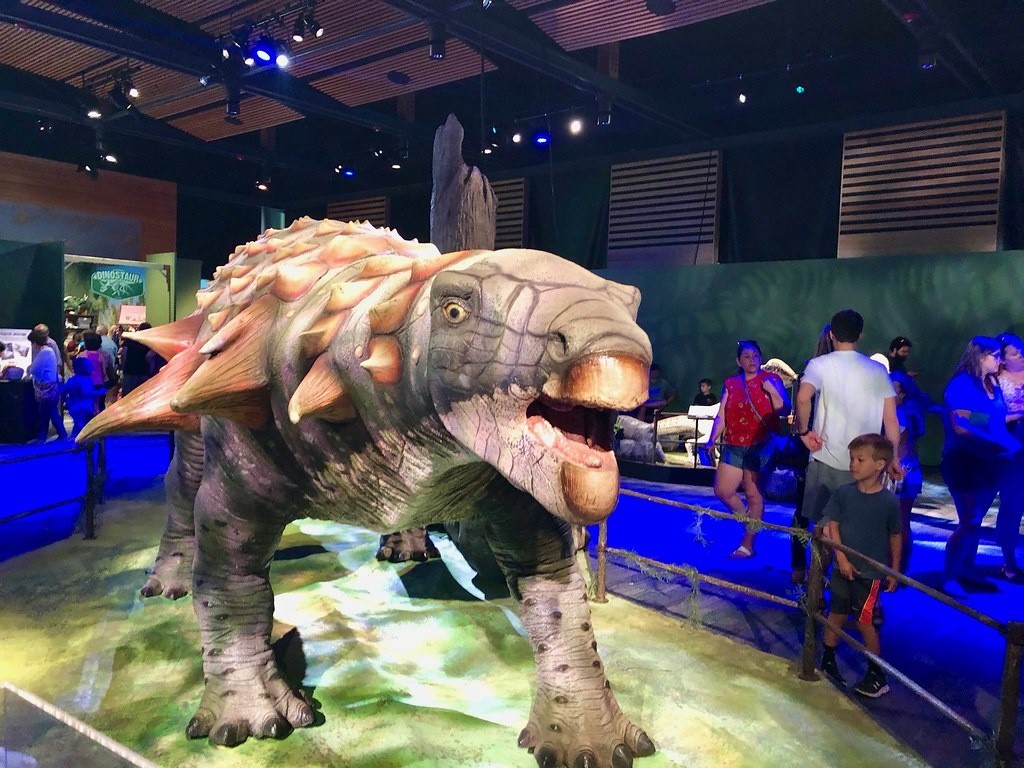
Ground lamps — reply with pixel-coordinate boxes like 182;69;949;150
107;80;132;111
428;22;446;60
199;10;290;115
292;13;304;42
303;8;324;38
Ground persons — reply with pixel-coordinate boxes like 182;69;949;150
798;307;901;627
0;322;173;445
693;378;717;406
886;336;920;378
940;334;1023;593
706;339;793;559
639;363;676;423
823;432;904;697
988;332;1024;584
885;372;942;589
789;322;836;592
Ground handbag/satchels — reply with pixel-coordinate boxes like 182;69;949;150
773;434;795;464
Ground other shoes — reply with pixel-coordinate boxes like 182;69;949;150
854;671;890;698
820;660;847;687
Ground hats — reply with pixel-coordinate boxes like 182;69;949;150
889;336;912;351
6;367;24;380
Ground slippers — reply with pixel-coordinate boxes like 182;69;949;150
733;546;753;558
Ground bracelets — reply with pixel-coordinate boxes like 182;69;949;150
797;428;810;436
